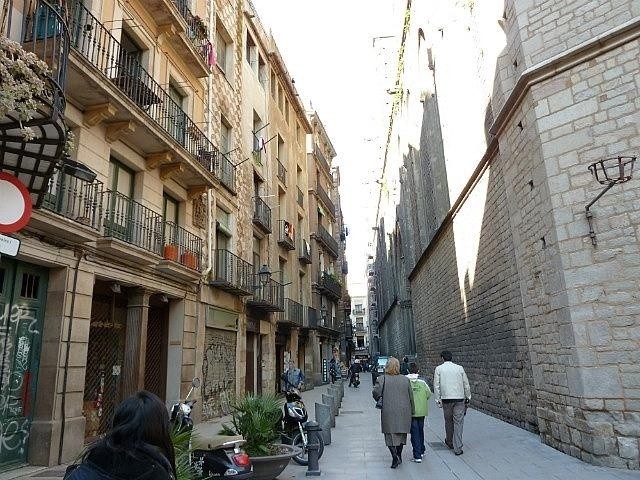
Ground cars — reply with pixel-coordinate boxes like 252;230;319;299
372;356;388;385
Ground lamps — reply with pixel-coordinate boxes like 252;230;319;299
317;305;327;320
251;264;271;293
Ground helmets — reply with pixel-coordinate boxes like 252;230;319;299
354;359;360;364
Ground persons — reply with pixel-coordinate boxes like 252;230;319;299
62;390;178;479
282;361;305;395
399;357;411;375
404;363;432;463
433;351;471;456
372;357;416;468
329;358;376;388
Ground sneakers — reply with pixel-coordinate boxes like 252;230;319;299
410;452;425;463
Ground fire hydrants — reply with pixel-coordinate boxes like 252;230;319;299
305;421;321;475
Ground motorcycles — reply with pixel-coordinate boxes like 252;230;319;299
170;377;255;479
272;381;324;465
350;371;361;387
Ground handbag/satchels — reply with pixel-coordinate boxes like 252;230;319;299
375;397;384;409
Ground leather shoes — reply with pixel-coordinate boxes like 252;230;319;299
391;453;402;468
445;438;463;455
